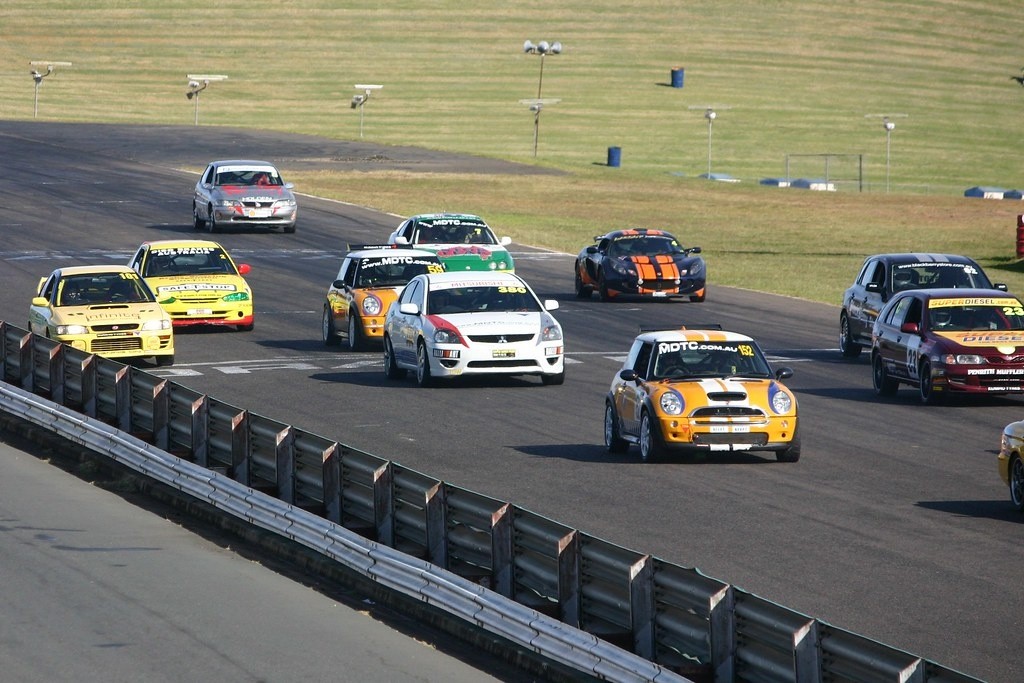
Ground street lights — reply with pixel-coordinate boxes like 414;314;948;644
705;111;716;180
523;39;561;156
883;122;897;194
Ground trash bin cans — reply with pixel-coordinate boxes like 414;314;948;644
607;146;622;166
671;67;685;88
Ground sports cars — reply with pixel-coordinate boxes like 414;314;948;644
575;227;707;303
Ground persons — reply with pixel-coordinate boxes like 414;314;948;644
221;173;232;182
429;291;451;311
422;229;442;242
254;173;271;185
658;351;686;374
62;283;80;302
894;268;912;286
152;255;171;269
617;239;632;251
929;308;955;329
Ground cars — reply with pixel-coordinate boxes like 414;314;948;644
321;244;449;352
839;252;1008;358
998;419;1024;513
28;264;175;366
383;270;565;388
870;287;1024;405
389;213;516;273
191;159;298;235
124;239;254;332
604;323;802;465
